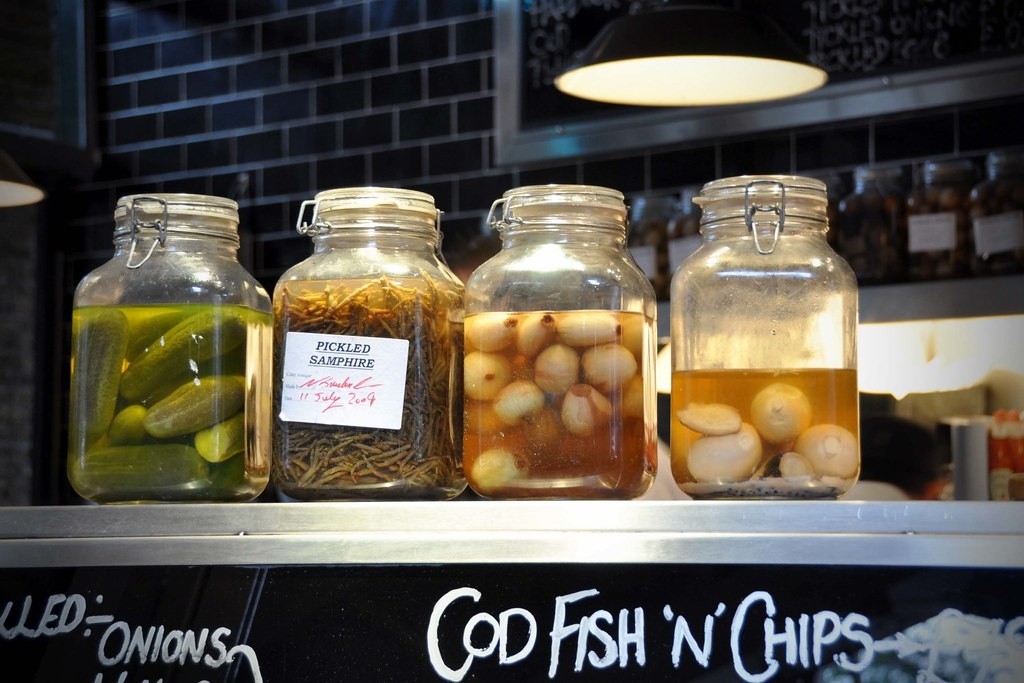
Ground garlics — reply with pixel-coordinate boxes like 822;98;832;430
462;310;645;493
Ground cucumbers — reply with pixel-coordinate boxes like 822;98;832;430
70;308;259;499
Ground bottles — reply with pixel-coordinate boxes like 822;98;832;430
461;183;658;498
67;193;273;503
668;176;861;498
475;151;1024;286
273;185;468;500
